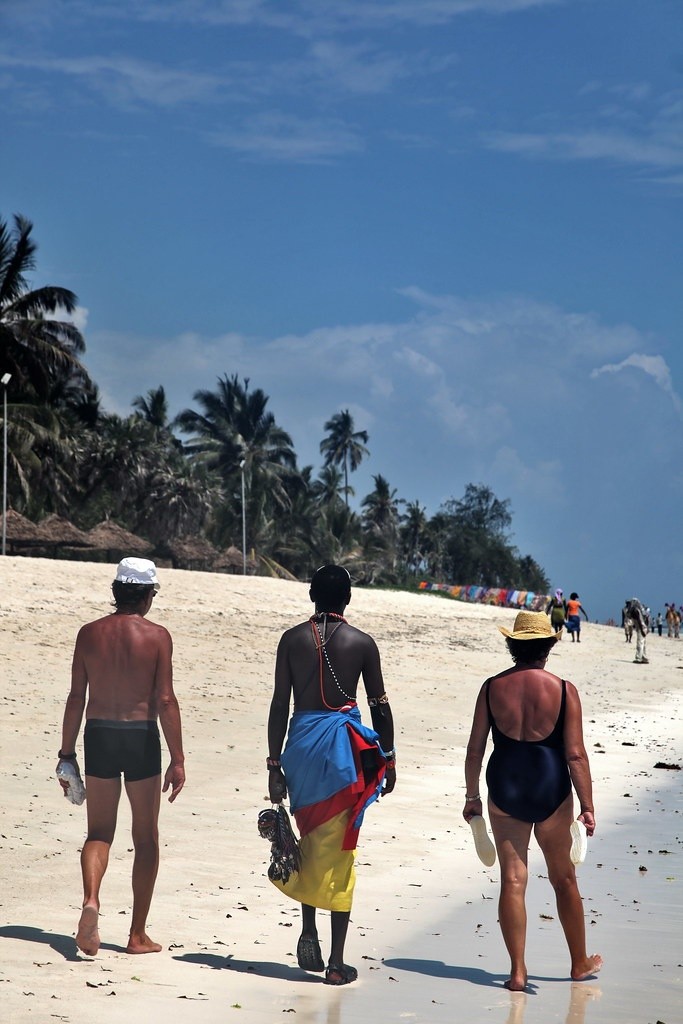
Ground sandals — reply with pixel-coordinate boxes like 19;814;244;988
297;930;325;972
325;964;358;985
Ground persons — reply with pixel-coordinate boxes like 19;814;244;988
266;566;396;985
462;612;603;989
546;589;588;642
622;598;683;663
608;617;614;626
56;557;186;956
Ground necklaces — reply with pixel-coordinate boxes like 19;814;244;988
517;664;541;669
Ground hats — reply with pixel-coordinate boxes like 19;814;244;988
115;557;161;590
498;612;564;640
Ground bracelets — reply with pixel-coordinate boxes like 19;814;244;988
465;793;480;801
266;757;282;770
385;748;396;769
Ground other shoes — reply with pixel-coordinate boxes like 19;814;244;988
569;820;587;867
470;815;496;867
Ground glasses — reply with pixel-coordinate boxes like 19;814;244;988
152;590;158;597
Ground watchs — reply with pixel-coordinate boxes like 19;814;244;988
58;749;77;759
